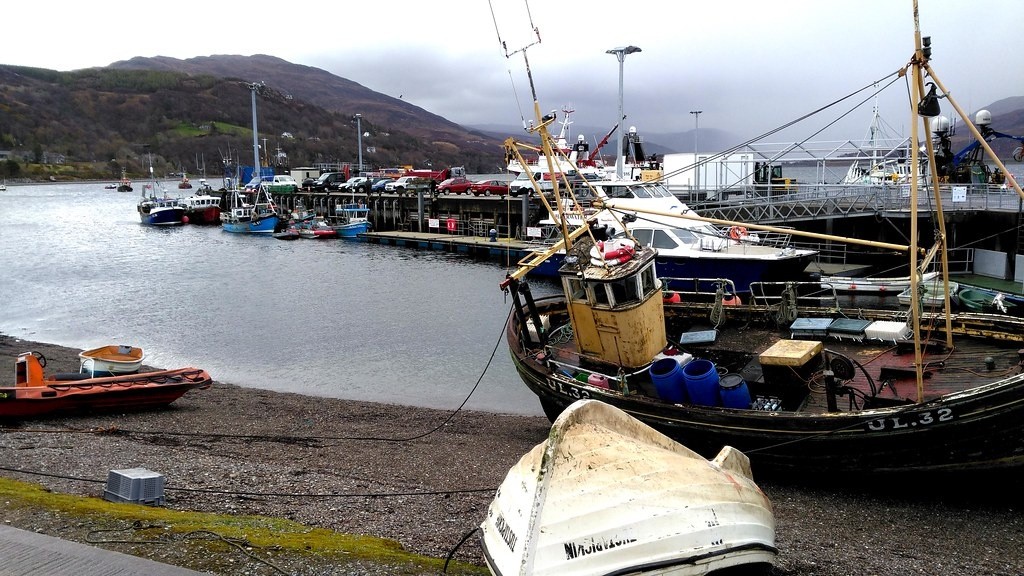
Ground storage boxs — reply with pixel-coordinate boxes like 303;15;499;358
103;466;166;507
788;317;908;341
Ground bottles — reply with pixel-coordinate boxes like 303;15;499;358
535;354;545;364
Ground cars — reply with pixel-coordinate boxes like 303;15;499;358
339;177;473;195
312;172;346;192
470;181;512;197
245;176;274;191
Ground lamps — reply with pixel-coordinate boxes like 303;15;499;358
917;82;951;119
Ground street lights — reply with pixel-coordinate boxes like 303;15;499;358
249;83;266;178
690;110;702;210
606;45;642;177
353;113;364;171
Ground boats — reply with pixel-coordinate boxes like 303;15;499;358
819;271;940;292
959;286;1017;314
137;151;188;227
522;181;821;301
117;166;133;192
78;344;145;377
844;81;1024;205
475;399;779;576
220;179;372;240
105;185;116;189
485;0;1024;481
0;351;214;420
185;194;222;227
178;168;193;189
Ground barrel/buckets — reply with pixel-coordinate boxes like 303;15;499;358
720;373;752;409
649;358;685;401
588;374;609;388
682;359;720;406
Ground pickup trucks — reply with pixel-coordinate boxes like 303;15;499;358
261;175;298;193
510;171;559;197
